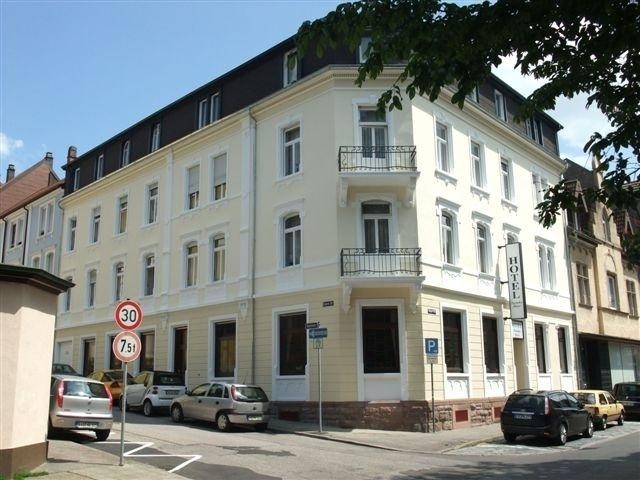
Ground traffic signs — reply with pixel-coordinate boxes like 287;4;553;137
309;328;328;339
114;301;144;332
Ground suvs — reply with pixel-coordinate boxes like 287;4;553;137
48;374;113;443
611;381;640;421
169;381;273;433
52;363;84;378
499;387;595;445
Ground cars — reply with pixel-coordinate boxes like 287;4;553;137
118;370;188;417
568;390;626;430
86;368;134;407
